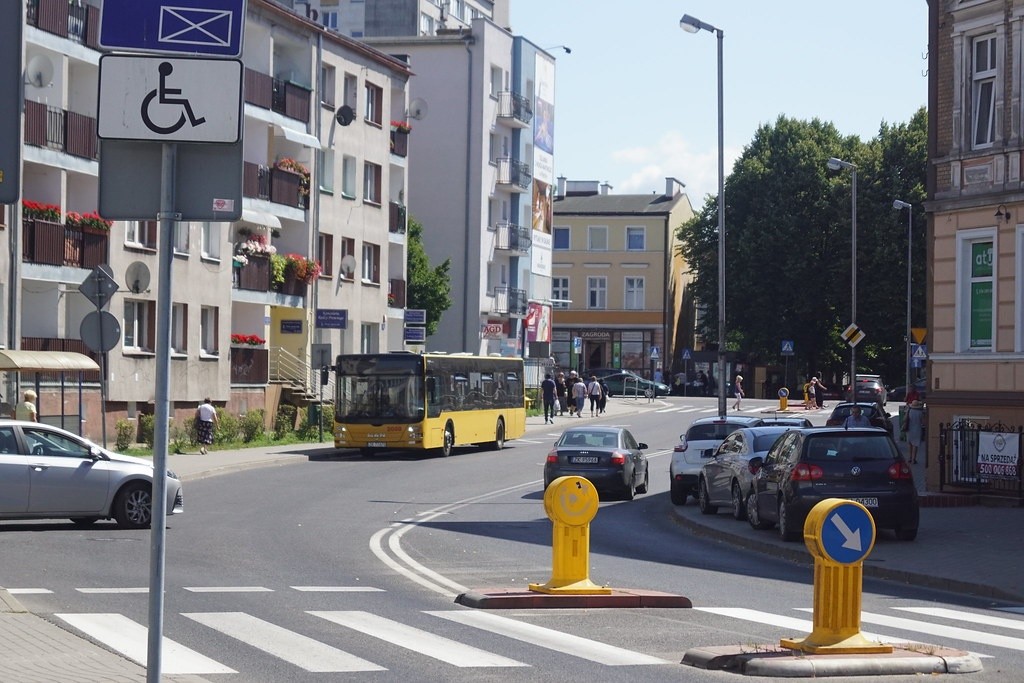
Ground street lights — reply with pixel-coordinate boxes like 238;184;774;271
890;198;912;395
826;158;858;402
679;15;728;419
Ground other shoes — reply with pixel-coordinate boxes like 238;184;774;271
554;409;606;418
549;419;554;425
544;421;549;425
200;447;207;454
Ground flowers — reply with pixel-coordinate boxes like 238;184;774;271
21;194;114;232
232;225;323;287
272;155;312;178
388;293;396;307
391;119;413;134
231;333;267;347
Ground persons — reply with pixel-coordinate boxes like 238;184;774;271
699;370;715;396
902;383;924;464
842;405;870;427
555;370;587;418
194;397;220;454
542;373;558;423
645;369;662;383
675;377;681;386
362;390;369;405
16;390;38;422
588;375;609;417
763;381;768;389
805;371;827;409
663;367;670;385
733;375;745;411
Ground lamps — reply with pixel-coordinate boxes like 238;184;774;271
993;204;1010;223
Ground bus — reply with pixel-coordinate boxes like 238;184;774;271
319;349;528;456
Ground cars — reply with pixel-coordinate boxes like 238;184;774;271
543;424;650;500
670;402;893;520
0;420;184;529
578;368;670;397
744;424;919;542
845;373;887;405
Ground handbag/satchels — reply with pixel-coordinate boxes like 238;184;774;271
553;399;561;411
901;409;910;432
588;392;593;398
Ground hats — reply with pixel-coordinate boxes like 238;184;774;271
812;376;819;382
909;399;923;408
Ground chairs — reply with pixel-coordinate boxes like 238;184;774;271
376;387;389;404
692;433;709;440
569;438;585;444
843;409;850;416
603;437;615;445
862;409;875;414
